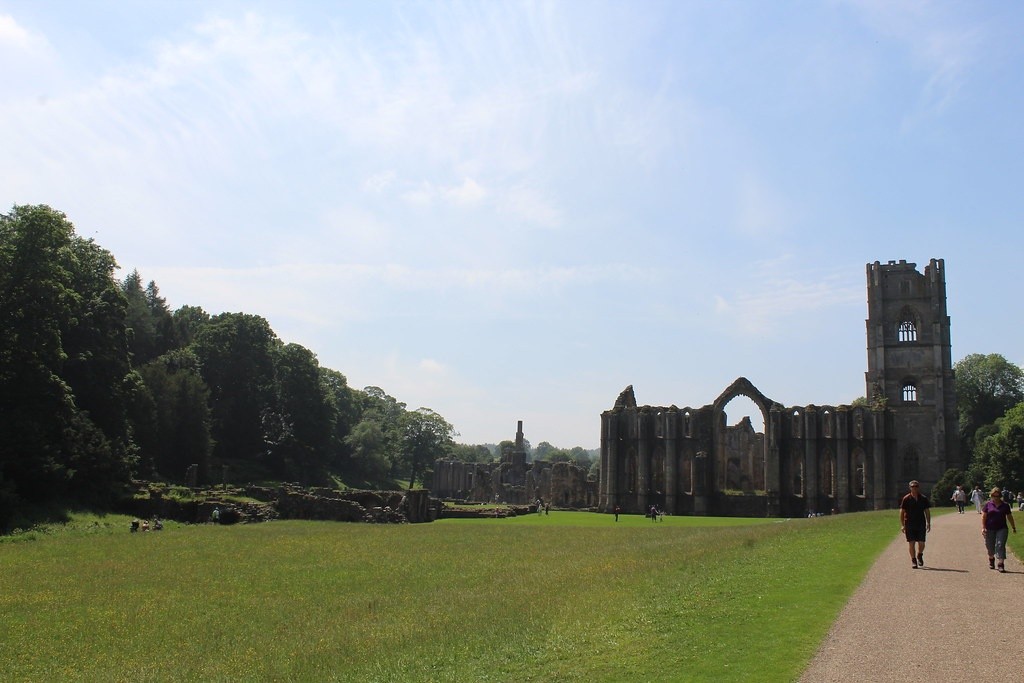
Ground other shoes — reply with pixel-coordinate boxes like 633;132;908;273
989;556;996;569
958;512;961;514
962;511;964;514
998;562;1004;572
917;553;923;566
911;558;918;568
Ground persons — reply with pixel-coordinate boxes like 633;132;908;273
970;486;984;514
614;506;620;521
1001;487;1024;512
651;504;664;523
808;507;836;519
495;506;502;518
212;507;220;525
899;480;931;569
130;518;164;533
537;503;550;518
980;486;1017;572
950;484;967;514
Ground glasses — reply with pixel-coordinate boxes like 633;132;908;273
911;486;918;487
992;495;1000;498
